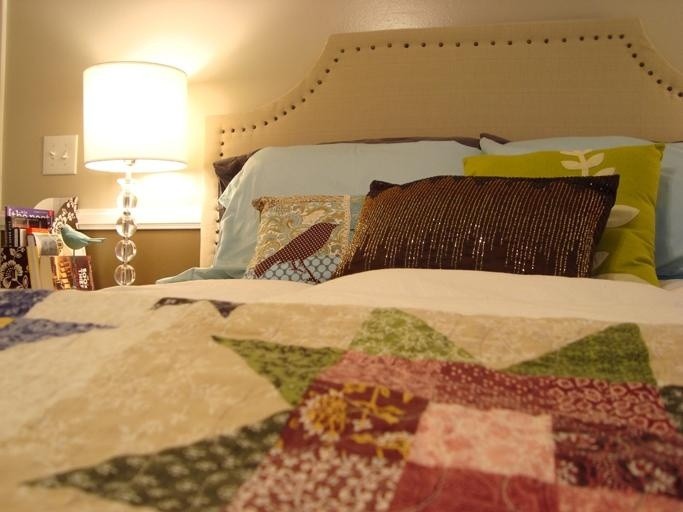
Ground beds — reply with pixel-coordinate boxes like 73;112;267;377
0;14;683;510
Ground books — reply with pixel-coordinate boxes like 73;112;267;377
1;204;97;292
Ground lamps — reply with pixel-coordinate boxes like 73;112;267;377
80;58;193;287
